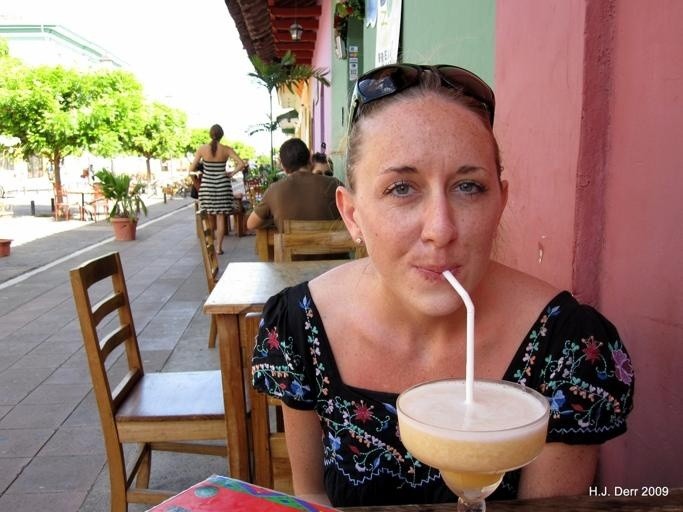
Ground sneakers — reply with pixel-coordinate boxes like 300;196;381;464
216;248;226;255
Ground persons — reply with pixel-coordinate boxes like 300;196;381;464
309;152;334;177
248;62;635;508
246;137;354;262
187;123;244;256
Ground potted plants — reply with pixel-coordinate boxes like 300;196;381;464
89;167;149;241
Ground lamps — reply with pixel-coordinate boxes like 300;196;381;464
287;0;304;43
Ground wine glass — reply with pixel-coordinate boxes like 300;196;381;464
394;376;554;512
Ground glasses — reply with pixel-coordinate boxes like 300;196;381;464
346;61;497;133
